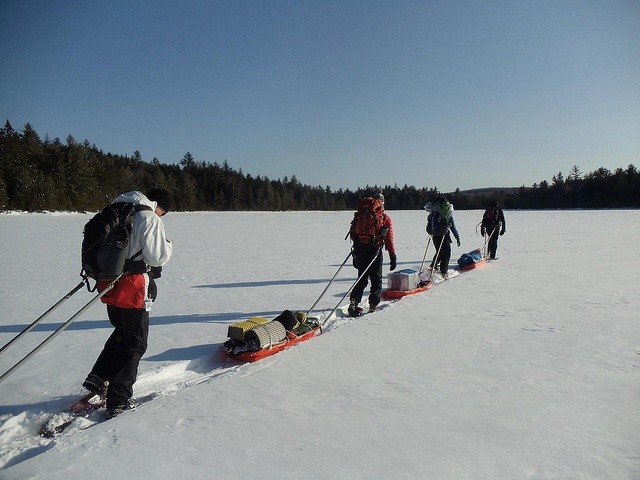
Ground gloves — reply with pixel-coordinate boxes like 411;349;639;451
481;227;486;237
457;237;460;246
499;230;505;236
389;254;396;272
148;272;157;303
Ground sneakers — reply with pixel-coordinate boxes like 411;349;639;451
369;304;382;312
348;307;363;317
105;400;141;419
84;372;108;398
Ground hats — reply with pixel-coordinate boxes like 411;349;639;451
147;189;170;217
374;189;385;205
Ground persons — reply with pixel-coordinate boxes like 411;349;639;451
429;197;460;277
348;193;396;317
82;190;173;418
480;201;505;259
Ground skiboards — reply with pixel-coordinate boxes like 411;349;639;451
40;391;108;438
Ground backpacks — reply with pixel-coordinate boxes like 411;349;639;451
345;197;385;248
79;203;153;293
426;211;449;235
484;209;499;226
